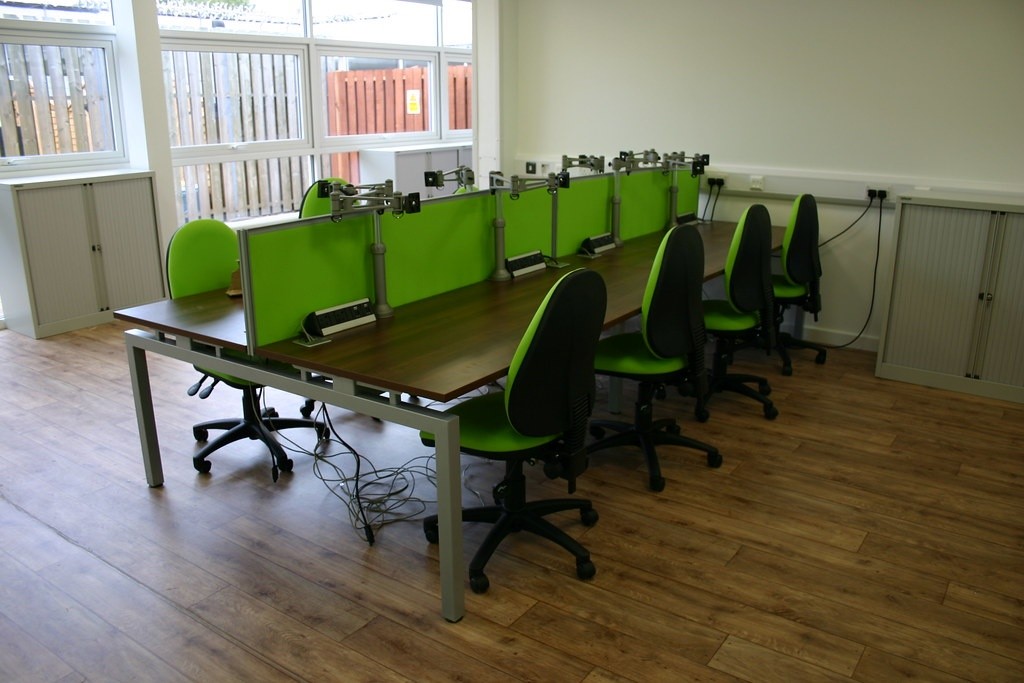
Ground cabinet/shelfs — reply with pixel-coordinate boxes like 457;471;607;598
875;193;1024;402
1;170;169;340
359;141;473;206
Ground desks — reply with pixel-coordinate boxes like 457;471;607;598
110;218;795;625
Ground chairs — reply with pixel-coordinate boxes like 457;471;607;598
723;191;828;378
454;184;479;195
299;177;359;219
420;264;610;595
547;224;722;493
651;201;784;424
164;219;332;473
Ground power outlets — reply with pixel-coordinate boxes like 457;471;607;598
704;173;727;191
750;175;763;190
864;183;890;200
